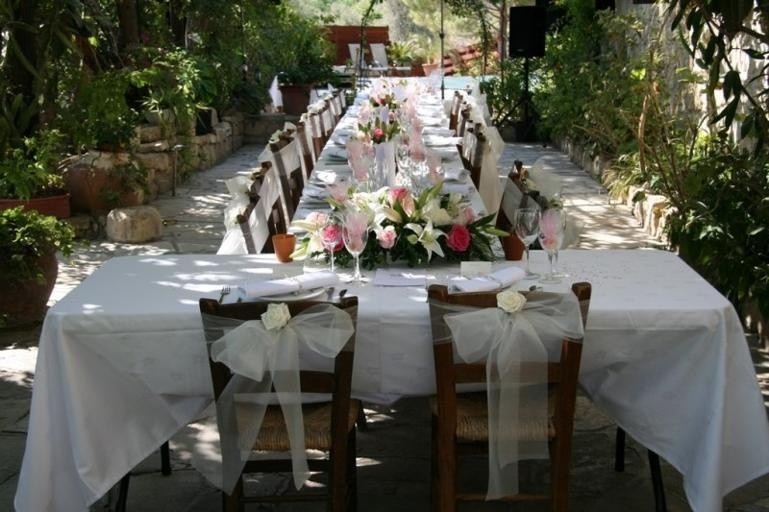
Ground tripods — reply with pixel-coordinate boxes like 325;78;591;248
496;57;552;148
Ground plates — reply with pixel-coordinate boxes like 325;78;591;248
247;272;326;302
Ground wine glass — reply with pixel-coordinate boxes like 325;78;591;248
342;218;370;287
515;207;541;280
316;212;342;277
538;209;570;285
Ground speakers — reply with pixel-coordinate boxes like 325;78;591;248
509;6;546;58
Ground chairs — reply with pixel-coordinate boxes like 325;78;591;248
426;281;593;512
345;42;410;76
196;297;361;510
234;87;567;255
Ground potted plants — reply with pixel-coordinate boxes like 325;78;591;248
260;16;340;114
1;67;148;329
420;51;440;77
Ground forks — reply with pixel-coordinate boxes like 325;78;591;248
218;285;230;305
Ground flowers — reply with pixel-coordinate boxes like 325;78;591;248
495;288;530;314
261;302;291;332
